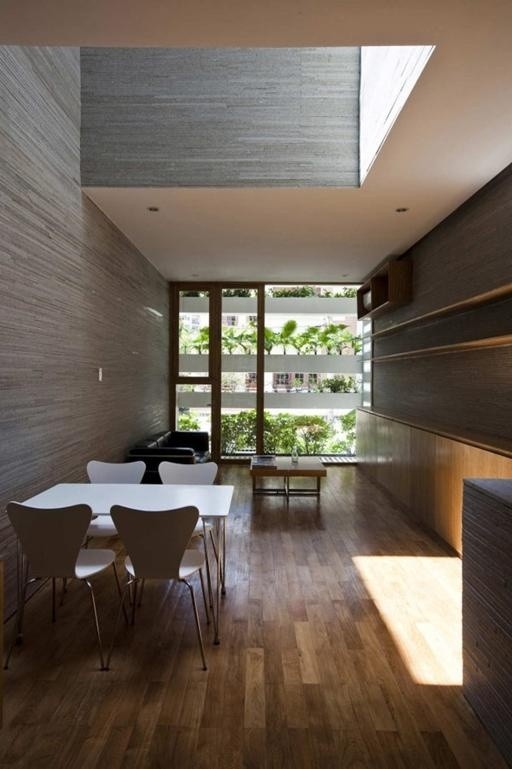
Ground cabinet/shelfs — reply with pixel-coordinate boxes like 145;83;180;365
462;478;512;769
357;259;412;321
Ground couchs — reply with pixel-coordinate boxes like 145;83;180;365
125;431;209;483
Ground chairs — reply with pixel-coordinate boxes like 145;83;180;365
86;461;146;627
6;501;121;672
104;505;208;671
159;461;226;608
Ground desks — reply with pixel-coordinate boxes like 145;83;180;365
19;483;235;645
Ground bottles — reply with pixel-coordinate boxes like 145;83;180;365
291;446;298;465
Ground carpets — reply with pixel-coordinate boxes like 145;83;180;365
351;556;464;687
